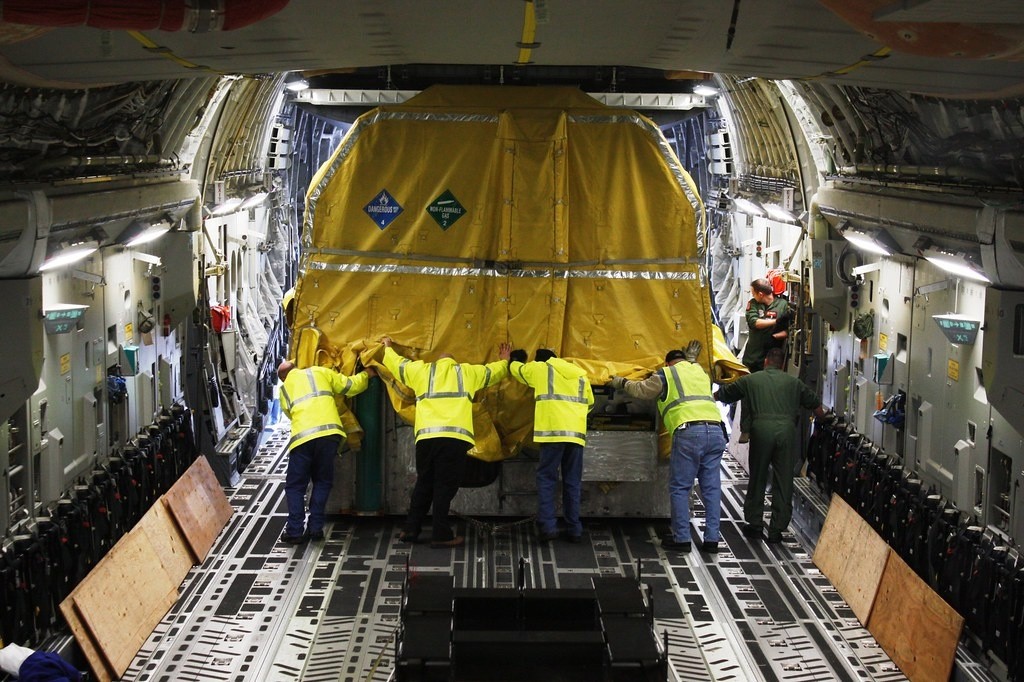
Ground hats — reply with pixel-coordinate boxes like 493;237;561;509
665;349;688;366
536;348;556;362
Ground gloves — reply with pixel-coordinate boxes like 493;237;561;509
510;349;527;364
682;341;702;363
605;376;625;390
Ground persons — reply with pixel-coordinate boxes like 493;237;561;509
713;279;823;544
382;337;513;548
508;347;595;543
277;361;378;542
605;340;727;553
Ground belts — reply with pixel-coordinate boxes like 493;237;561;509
675;421;720;429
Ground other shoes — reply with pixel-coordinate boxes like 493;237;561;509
281;533;302;543
562;530;581;543
743;524;762;537
309;530;322;540
769;534;782;542
703;540;718;553
400;530;418;540
739;433;750;443
539;532;559;541
661;538;691;553
430;535;463;549
